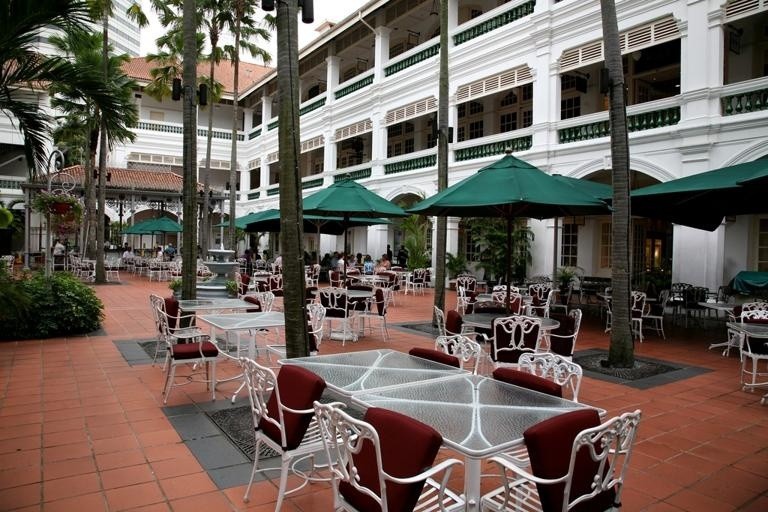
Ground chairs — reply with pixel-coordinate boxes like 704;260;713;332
0;241;147;285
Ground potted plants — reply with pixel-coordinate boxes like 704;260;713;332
28;188;87;235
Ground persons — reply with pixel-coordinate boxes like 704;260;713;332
197;244;282;270
104;242;184;260
304;244;409;273
53;235;66;264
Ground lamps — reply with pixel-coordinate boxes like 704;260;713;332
260;1;316;25
197;191;213;199
170;76;208;108
598;65;612;97
431;121;455;143
90;168;113;185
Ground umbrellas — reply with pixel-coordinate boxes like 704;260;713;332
212;173;412;287
405;152;612;316
630;153;768;231
119;218;146;249
139;215;183;249
127;219;163;248
549;174;612;288
